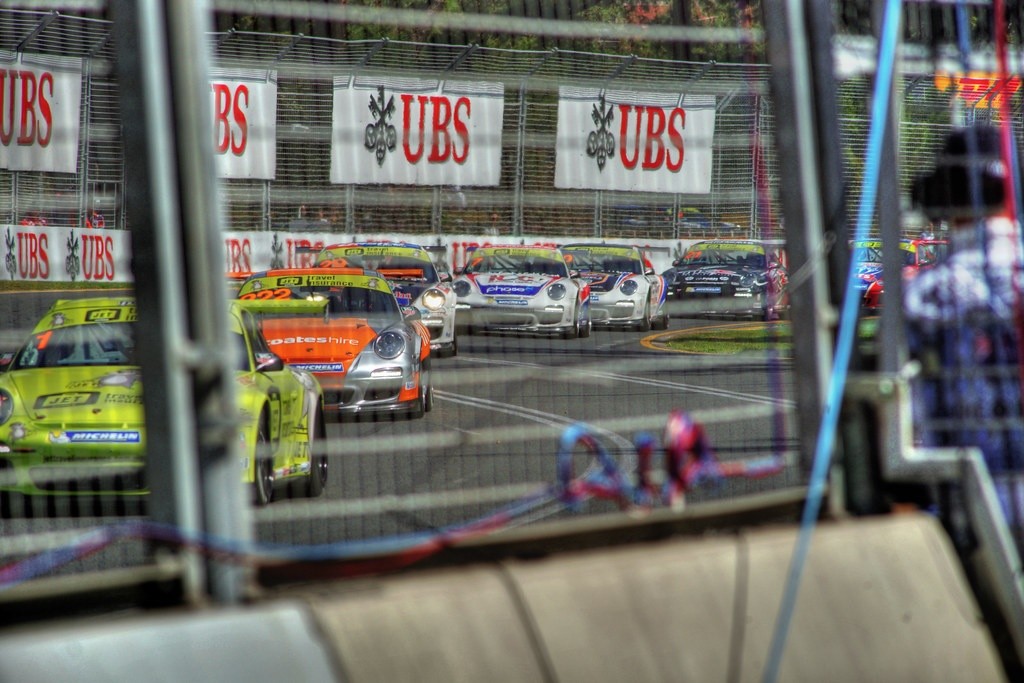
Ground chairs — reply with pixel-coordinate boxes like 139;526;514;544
602;259;622;271
341;287;372;313
747;252;761;264
533;258;557;273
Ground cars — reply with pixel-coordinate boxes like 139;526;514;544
659;231;950;322
0;294;331;506
237;269;436;417
452;245;595;340
312;241;458;358
559;244;668;331
